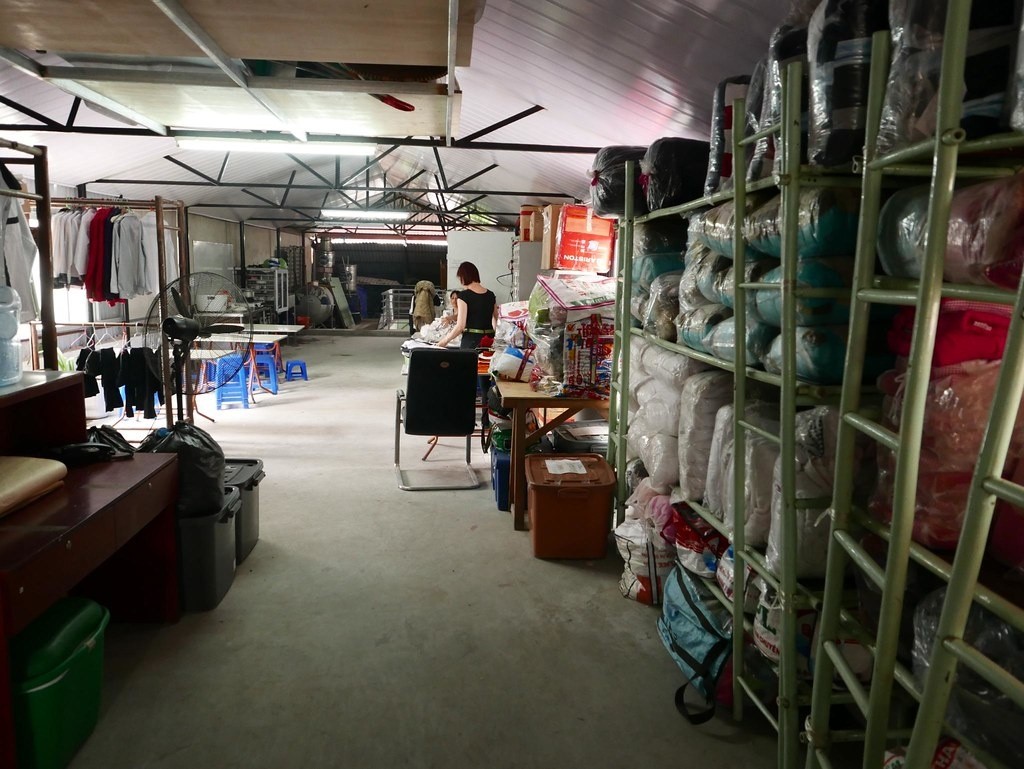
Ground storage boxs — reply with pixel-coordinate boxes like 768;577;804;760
540;204;561;269
223;456;266;566
520;204;547;242
529;212;544;242
524;452;615;560
490;443;527;512
552;418;610;458
1;598;110;769
553;204;642;275
173;484;243;612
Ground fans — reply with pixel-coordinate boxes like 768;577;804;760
141;271;254;422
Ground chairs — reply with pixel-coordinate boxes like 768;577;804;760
393;347;478;492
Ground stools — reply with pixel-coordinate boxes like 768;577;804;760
254;343;282;377
285;360;307;381
248;355;278;395
215;356;250;409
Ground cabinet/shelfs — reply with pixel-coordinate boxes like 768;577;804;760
606;1;1023;769
247;268;289;313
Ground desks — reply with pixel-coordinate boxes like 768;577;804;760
494;377;610;532
0;371;179;767
192;307;271;325
149;322;306;394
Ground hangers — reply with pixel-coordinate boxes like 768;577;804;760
94;322;119;350
87;322;103;351
70;322;94;349
63;196;134;215
121;322;154;349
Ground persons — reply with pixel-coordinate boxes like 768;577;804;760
437;261;499;432
409;285;461;336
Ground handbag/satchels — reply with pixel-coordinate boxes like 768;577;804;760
613;504;672;602
656;558;744;693
